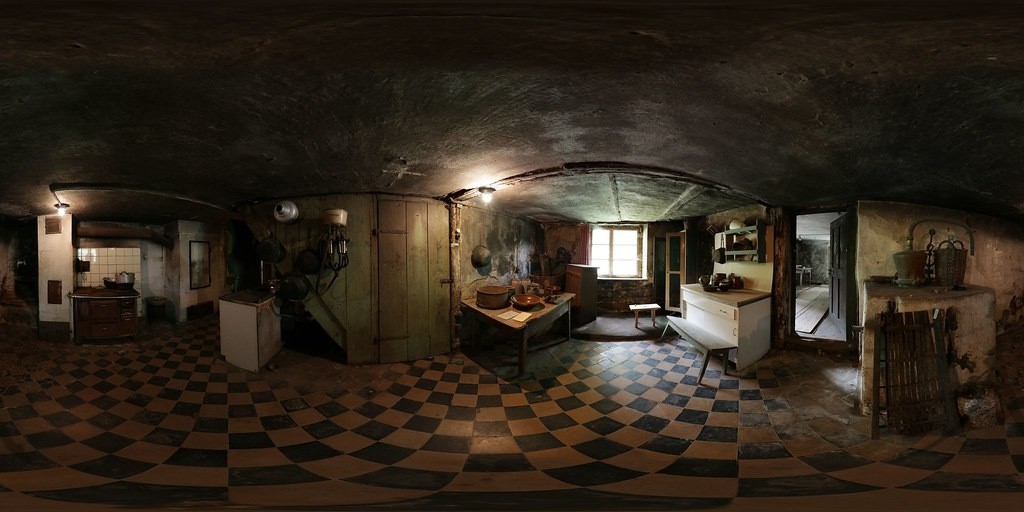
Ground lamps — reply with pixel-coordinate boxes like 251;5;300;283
479;187;496;205
55;204;70;216
799;235;801;241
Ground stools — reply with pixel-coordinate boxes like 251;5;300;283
629;303;661;327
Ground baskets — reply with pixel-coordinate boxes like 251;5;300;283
934;240;968;286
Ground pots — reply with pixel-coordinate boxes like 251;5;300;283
103;278;116;288
117;283;134;290
477;286;509;309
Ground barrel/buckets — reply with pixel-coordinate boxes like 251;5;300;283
729;218;746;234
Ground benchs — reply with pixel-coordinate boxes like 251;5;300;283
659;314;737;385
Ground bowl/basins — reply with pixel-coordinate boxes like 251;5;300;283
511;294;540;308
702;285;718;292
471;245;491;268
548;286;561;294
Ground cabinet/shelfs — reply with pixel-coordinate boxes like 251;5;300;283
652;229;697;313
567;264;597;327
72;287;141;343
218;291;282;375
681;283;771;372
724;218;766;263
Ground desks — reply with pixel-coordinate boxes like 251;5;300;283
461;292;576;376
797;267;813;288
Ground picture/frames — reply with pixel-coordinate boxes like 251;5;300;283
189;240;211;290
